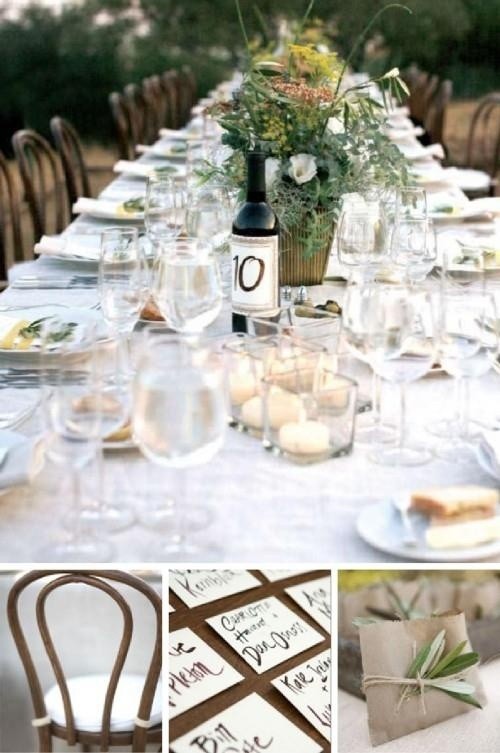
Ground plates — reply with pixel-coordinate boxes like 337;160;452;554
424;201;470;223
0;303;83;360
89;211;144;222
45;251;136;266
358;483;499;560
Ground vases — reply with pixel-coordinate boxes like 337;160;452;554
264;207;335;284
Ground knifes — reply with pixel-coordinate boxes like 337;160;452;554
11;273;129;289
0;367;89;390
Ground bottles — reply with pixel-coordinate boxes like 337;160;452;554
231;151;281;335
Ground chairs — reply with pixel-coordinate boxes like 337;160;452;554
50;116;92;223
110;92;134;160
466;95;499;199
408;76;439;127
12;130;67;260
417;81;452;161
7;569;161;752
165;69;182;128
143;76;168;143
124;84;146;158
409;72;427;114
0;155;24;290
182;63;198;121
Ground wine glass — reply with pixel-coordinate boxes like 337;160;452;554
396;189;438;284
436;290;498;446
153;241;224;337
132;337;230;562
41;324;132;559
100;231;147;341
390;219;436;284
143;140;234;242
336;209;389;326
342;266;394;445
443;249;485;293
366;286;439;468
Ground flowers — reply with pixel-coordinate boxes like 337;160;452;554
206;1;411;258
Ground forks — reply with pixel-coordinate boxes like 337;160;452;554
387;485;418;547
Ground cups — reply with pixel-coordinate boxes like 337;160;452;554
246;305;341;367
260;370;358;465
227;333;327;439
